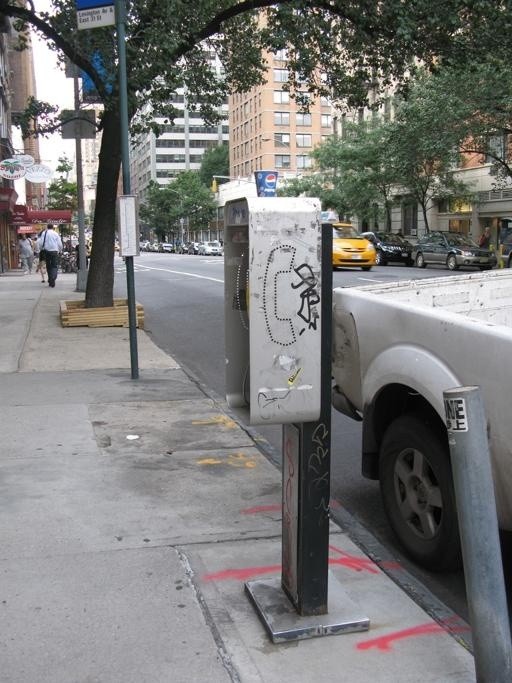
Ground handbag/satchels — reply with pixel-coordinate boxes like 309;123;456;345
39;249;48;261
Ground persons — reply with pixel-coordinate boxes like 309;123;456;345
479;225;492;250
17;231;90;283
37;222;65;288
395;228;404;239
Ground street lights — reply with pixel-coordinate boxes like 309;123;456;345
262;137;290;150
158;186;185;243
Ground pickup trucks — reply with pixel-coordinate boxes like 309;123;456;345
330;268;512;570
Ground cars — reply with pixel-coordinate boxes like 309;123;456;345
139;239;223;256
332;222;377;271
497;227;512;268
362;231;414;268
410;230;498;270
66;236;120;255
